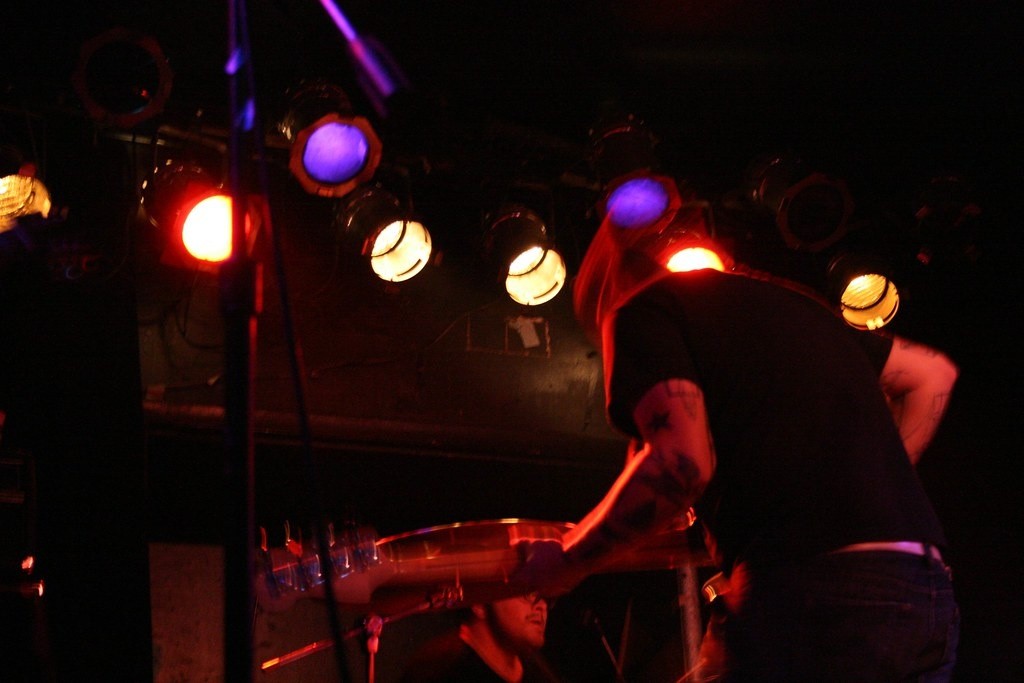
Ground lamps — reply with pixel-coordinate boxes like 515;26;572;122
0;23;982;334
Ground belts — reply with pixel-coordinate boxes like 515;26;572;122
831;541;944;560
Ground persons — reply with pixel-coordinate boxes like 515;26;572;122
395;186;962;682
